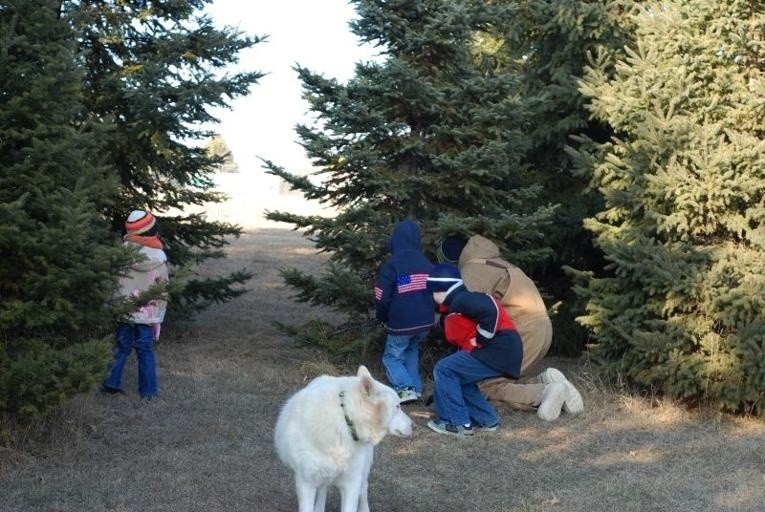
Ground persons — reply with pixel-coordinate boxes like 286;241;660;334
98;210;169;399
374;220;434;404
426;265;523;436
435;230;584;422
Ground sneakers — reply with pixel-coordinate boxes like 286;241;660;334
471;423;500;433
538;367;584;414
397;386;417;405
531;383;568;421
100;385;124;396
425;416;474;440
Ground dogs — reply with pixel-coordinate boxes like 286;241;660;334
272;364;417;512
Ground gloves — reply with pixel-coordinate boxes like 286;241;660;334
154;324;160;342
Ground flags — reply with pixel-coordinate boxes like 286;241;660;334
397;273;429;294
374;288;382;302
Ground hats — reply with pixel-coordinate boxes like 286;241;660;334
125;210;156;235
426;264;462;291
436;232;467;267
